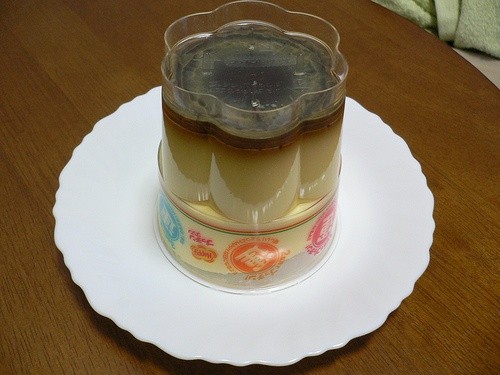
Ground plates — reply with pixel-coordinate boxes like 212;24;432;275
52;85;436;366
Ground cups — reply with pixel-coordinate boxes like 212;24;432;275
158;1;350;287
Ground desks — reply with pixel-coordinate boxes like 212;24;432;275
0;0;500;375
